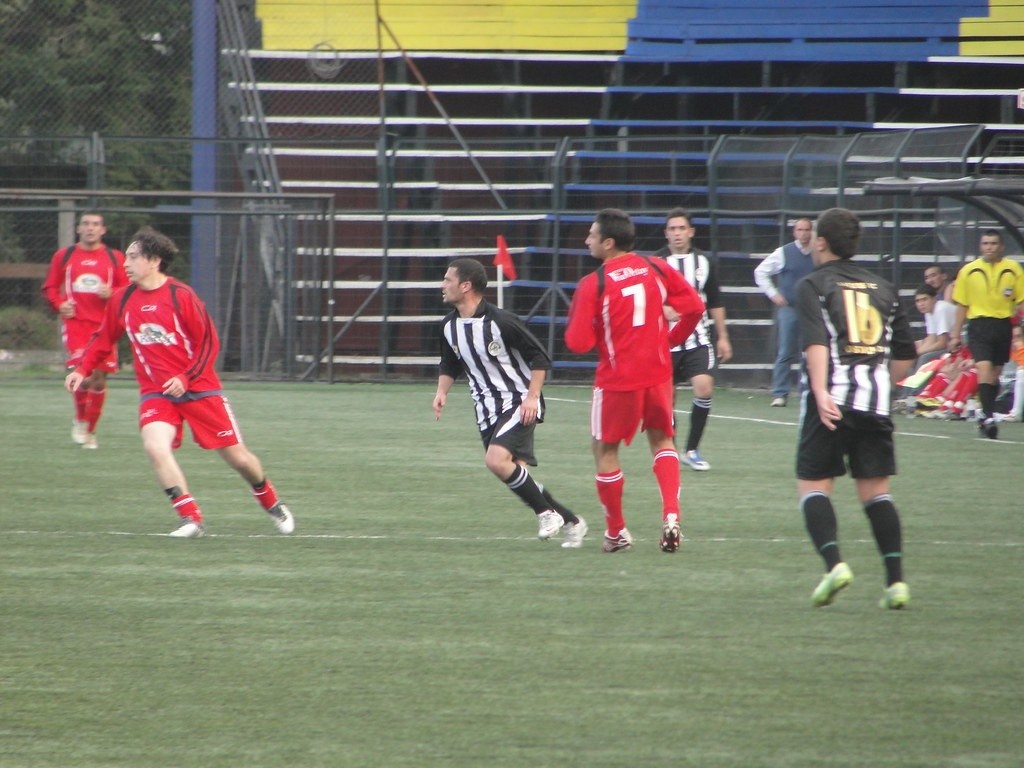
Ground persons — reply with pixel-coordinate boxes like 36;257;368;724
647;211;732;473
755;216;815;408
63;227;294;538
792;207;917;609
433;257;588;547
562;209;707;553
897;230;1024;442
42;207;130;449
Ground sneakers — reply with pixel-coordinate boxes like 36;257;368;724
878;581;910;609
809;561;855;607
562;514;589;549
168;519;208;538
601;526;634;552
537;507;566;541
264;500;295;534
680;448;712;472
71;419;89;444
81;432;98;449
661;513;684;553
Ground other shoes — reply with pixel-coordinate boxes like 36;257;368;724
770;396;787;406
891;394;1024;438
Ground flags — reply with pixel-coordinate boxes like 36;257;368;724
493;234;515;280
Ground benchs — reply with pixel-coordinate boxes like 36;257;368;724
218;0;1024;383
